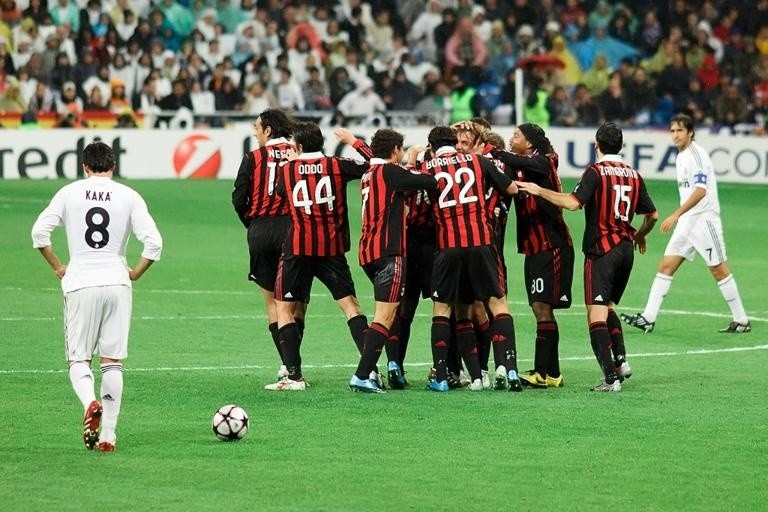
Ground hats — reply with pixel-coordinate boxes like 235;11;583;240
63;81;76;91
111;79;123;88
164;48;175;59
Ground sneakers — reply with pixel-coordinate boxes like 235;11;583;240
428;367;461;389
481;371;490;390
719;321;751;332
468;379;483;391
493;365;508;390
450;370;460;382
460;370;471;386
277;365;311;387
519;371;548;388
594;378;621;392
265;377;306;392
349;374;386;395
429;378;448;391
620;313;655;333
613;354;632;384
99;437;115;451
388;360;404;389
402;373;407;384
83;401;102;449
369;371;385;389
546;374;564;388
508;370;522;392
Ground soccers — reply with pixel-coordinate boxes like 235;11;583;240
211;405;249;441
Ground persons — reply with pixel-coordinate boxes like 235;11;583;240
232;109;305;378
399;148;458;388
264;124;385;392
623;112;746;334
33;144;163;452
448;120;489;387
454;130;507;389
516;123;656;391
491;125;575;387
0;1;767;126
348;129;436;395
407;127;520;391
